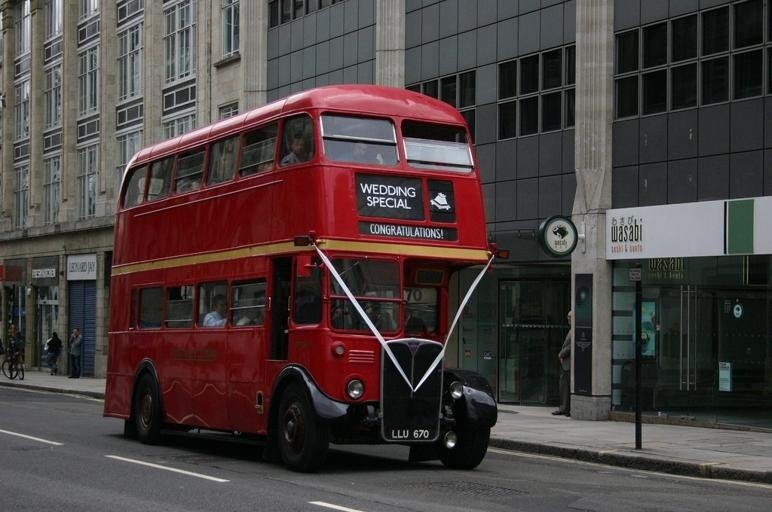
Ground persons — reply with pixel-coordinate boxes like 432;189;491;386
67;327;83;378
553;310;573;417
152;118;398;200
44;331;64;376
137;256;430;359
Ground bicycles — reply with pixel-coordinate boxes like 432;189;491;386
1;348;25;380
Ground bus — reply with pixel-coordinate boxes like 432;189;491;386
103;83;509;474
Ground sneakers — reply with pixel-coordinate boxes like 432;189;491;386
552;410;567;415
